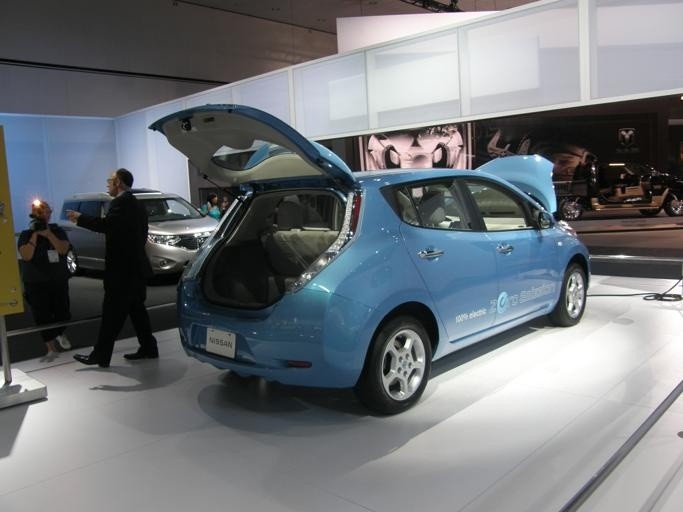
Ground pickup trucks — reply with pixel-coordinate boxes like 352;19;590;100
554;160;683;222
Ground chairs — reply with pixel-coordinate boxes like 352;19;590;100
418;191;445;229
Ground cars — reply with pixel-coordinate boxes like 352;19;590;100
147;105;591;416
55;189;221;280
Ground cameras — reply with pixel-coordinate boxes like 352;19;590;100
29;214;47;233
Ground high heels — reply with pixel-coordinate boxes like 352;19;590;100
73;354;110;368
123;345;158;360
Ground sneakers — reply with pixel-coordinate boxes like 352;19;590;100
40;351;59;363
56;335;72;350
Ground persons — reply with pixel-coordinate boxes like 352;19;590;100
206;193;220;219
16;193;78;358
219;196;229;215
569;151;597;211
63;166;159;369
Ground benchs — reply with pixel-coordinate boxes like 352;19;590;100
273;230;345;276
